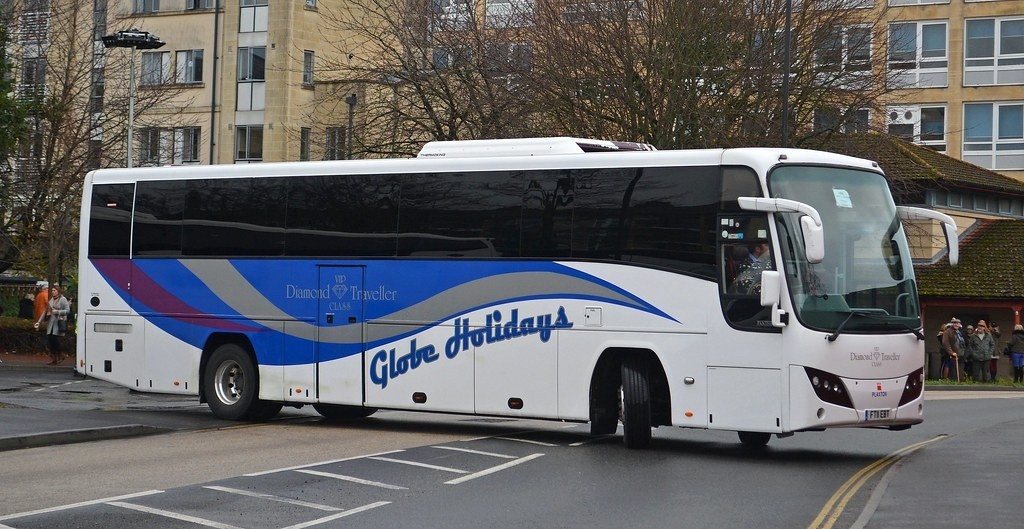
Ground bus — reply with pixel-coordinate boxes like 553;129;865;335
76;137;959;448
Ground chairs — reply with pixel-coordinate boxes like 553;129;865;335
729;242;750;279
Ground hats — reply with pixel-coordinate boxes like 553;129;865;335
978;320;986;326
41;282;48;289
967;325;974;330
951;318;961;322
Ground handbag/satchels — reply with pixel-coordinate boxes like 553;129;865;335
57;310;67;332
1004;343;1010;354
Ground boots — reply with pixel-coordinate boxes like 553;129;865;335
1013;366;1019;383
1019;365;1024;383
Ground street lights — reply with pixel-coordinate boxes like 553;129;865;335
102;29;168;167
345;94;357;160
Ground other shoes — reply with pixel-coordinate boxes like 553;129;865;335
47;352;57;365
56;351;65;364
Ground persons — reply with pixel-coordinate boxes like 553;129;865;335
34;283;48;322
736;243;770;296
1006;324;1024;383
18;293;35;319
65;296;74;321
34;285;70;365
936;317;1002;383
